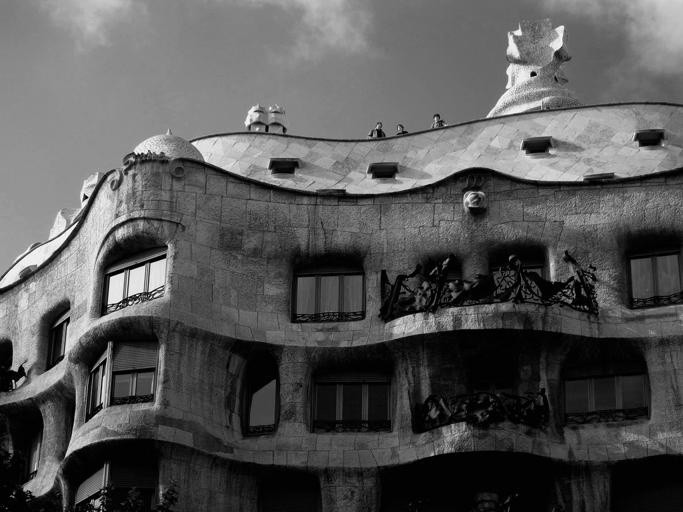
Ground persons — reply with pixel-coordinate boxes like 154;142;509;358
430;114;448;128
368;121;386;140
396;124;407;135
